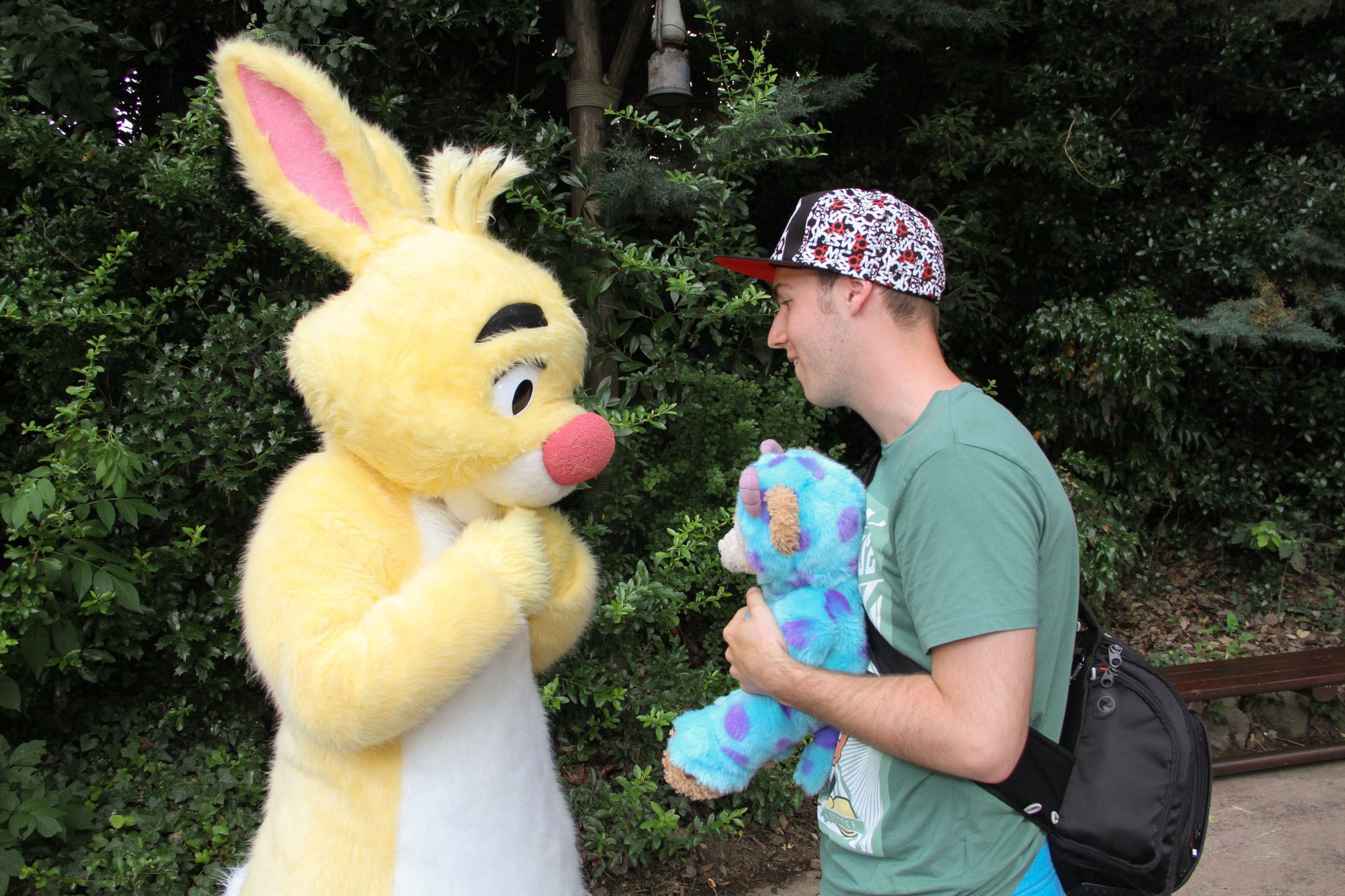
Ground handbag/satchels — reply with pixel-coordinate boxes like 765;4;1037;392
1043;627;1212;896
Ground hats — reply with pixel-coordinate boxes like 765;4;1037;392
713;188;945;303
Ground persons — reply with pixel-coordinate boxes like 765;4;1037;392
711;187;1080;896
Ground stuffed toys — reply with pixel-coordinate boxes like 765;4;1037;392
664;438;868;800
202;32;615;896
